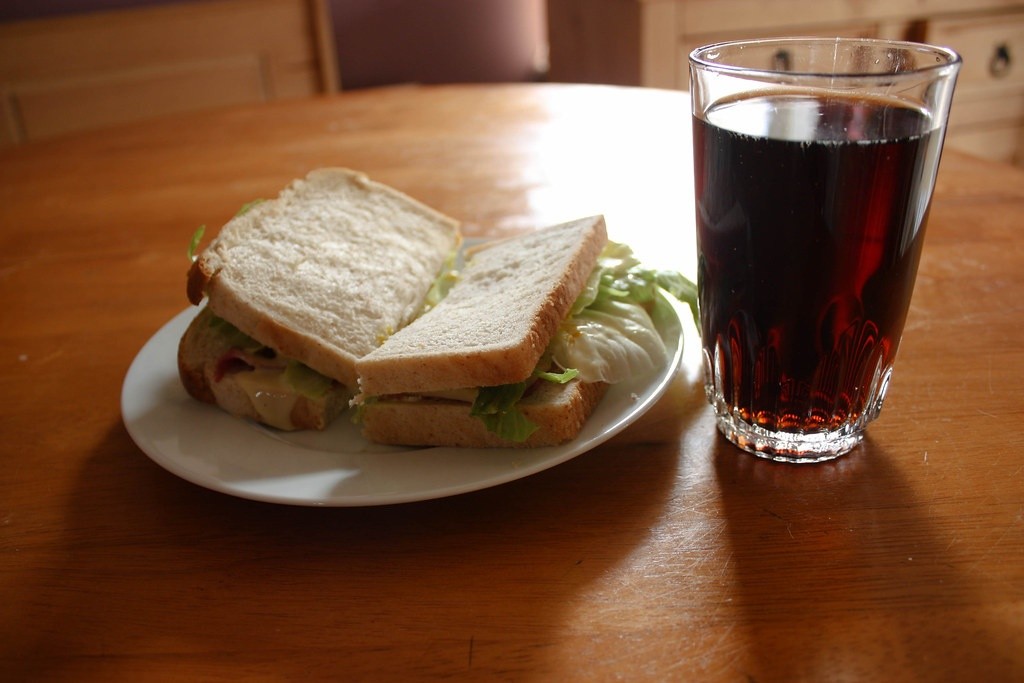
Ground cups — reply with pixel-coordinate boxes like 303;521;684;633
688;38;963;466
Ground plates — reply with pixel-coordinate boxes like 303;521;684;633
119;239;684;509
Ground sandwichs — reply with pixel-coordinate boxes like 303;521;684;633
177;166;460;435
345;214;701;451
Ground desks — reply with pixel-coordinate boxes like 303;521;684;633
0;82;1024;683
